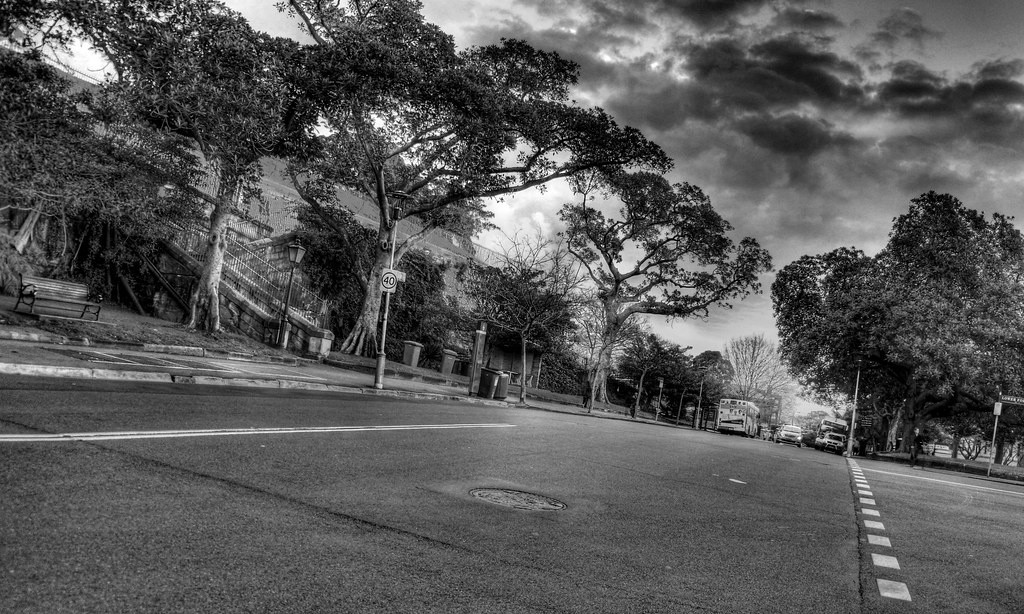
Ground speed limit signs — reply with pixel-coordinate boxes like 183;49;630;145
379;267;399;294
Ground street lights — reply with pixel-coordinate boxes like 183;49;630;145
846;350;869;458
987;380;1009;476
373;190;418;391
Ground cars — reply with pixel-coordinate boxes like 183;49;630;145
821;432;848;456
775;424;805;448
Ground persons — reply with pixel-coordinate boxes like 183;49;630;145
581;380;592;408
853;436;868;457
630;402;636;418
908;427;923;467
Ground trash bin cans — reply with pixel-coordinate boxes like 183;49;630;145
404;341;423;367
478;367;501;399
440;349;458;376
493;374;509;400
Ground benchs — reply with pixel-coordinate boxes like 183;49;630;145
14;272;101;321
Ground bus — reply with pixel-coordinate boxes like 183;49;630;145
814;415;848;451
718;398;760;439
